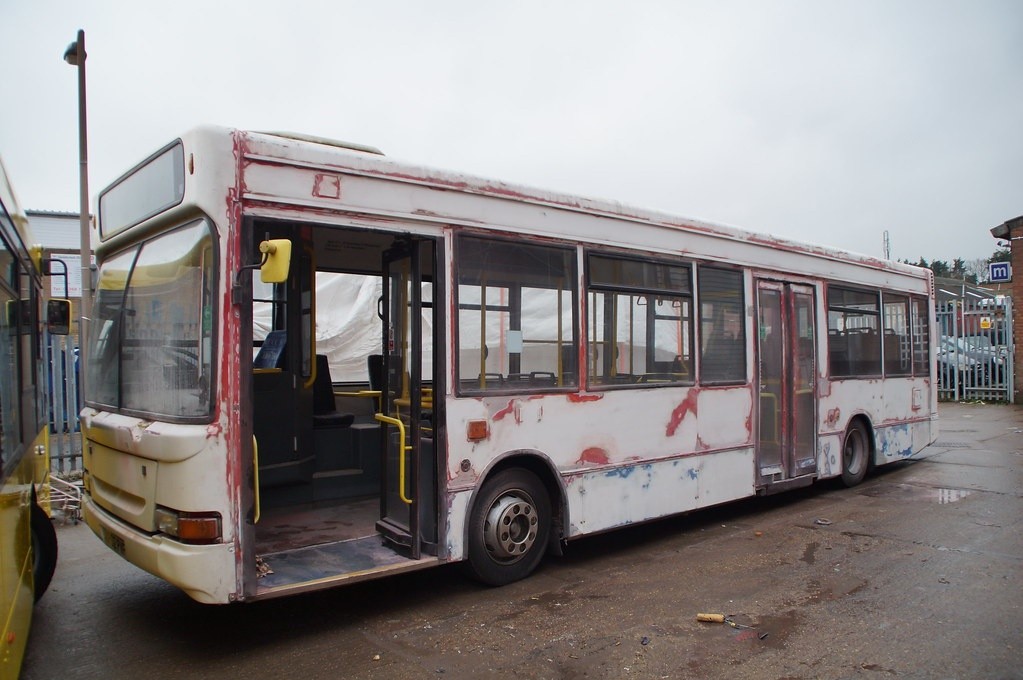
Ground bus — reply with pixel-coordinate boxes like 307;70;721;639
0;138;76;679
73;127;943;606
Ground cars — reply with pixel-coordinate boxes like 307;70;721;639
936;346;987;396
940;334;1006;383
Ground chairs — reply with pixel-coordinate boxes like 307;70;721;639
477;371;636;389
829;327;901;377
255;331;431;428
